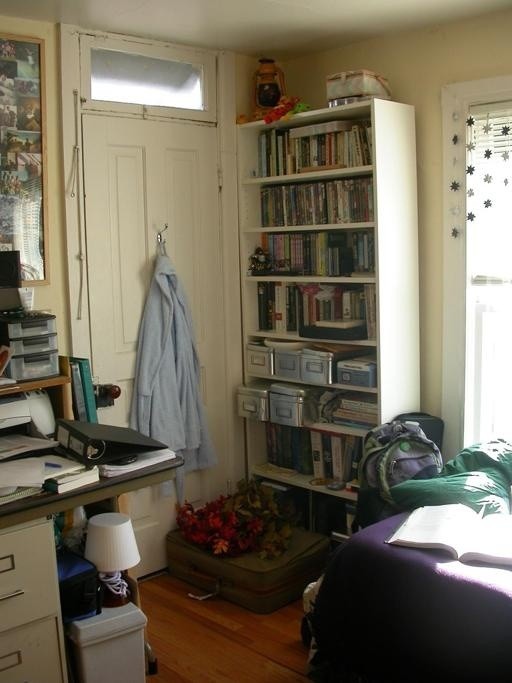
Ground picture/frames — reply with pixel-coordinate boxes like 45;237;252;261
0;33;52;289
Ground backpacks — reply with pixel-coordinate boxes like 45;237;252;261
350;418;446;534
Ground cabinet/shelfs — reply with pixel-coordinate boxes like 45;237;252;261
0;513;71;681
234;99;421;558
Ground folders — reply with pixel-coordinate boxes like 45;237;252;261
54;417;168;470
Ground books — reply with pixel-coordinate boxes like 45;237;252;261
0;465;100;506
383;504;512;565
258;121;376;342
253;396;378;493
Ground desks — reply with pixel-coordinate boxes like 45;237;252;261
0;424;186;682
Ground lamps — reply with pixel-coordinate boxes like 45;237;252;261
83;512;142;608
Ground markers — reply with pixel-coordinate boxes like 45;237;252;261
45;462;63;467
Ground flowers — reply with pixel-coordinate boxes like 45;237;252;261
174;472;299;564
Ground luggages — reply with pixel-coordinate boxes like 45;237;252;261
165;511;331;616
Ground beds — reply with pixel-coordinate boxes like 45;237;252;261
302;438;511;683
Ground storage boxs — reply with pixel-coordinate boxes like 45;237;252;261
325;68;392;99
236;344;377;428
56;547;98;623
